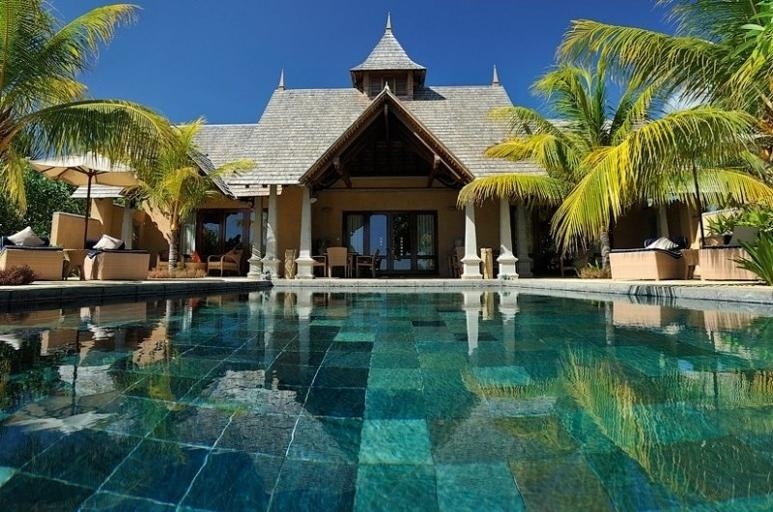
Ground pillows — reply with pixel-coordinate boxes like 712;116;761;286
6;226;123;251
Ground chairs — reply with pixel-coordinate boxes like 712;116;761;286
311;246;379;278
155;248;244;276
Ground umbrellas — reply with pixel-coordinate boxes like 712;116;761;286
27;149;142;252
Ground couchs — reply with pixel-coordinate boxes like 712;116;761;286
608;227;762;280
0;238;150;281
